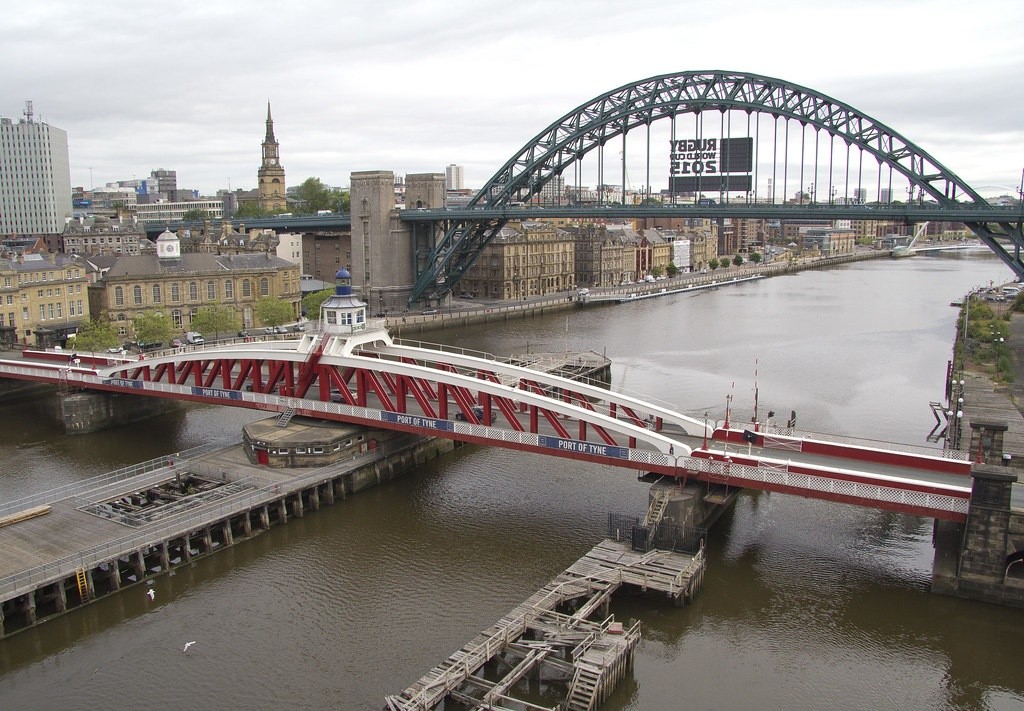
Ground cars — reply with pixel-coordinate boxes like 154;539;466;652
455;405;497;422
264;324;288;334
374;312;388;317
422;308;437;315
246;381;276;393
108;339;183;354
293;323;306;331
995;282;1024;302
329;388;358;403
237;331;250;337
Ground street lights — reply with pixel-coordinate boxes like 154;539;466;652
976;425;986;464
807;183;815;203
638;185;647;205
831;185;837;204
725;458;733;498
948;324;1005;449
723;393;730;429
701;412;710;451
707;455;713;494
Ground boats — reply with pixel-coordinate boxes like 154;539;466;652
891;246;917;257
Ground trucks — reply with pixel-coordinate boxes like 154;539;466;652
187;331;205;345
